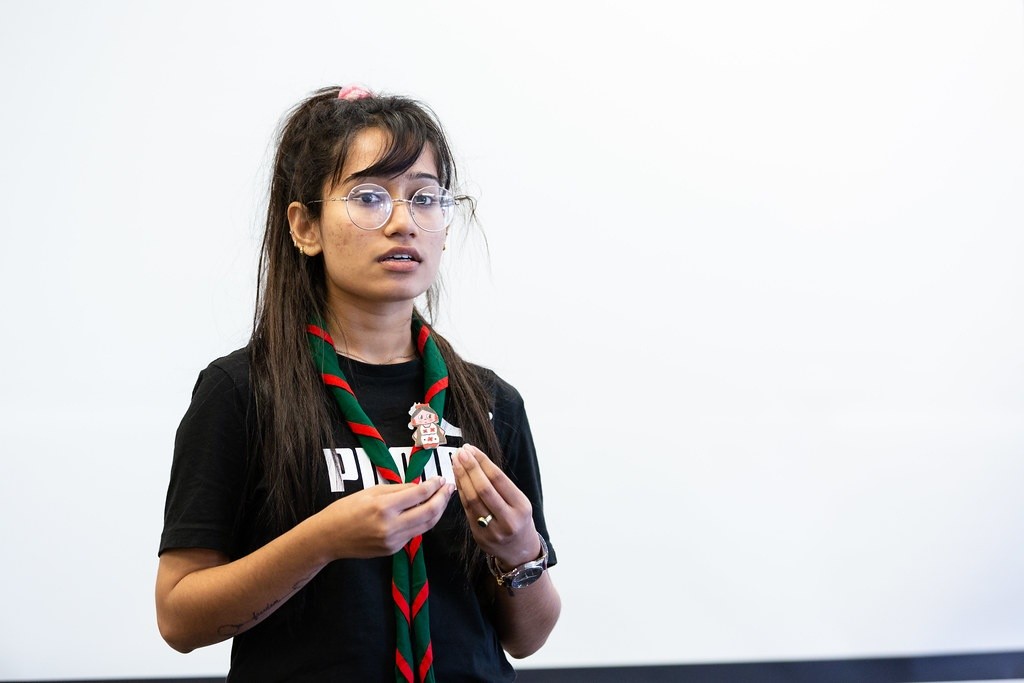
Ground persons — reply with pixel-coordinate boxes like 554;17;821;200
156;86;563;683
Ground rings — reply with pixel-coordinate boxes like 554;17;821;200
476;515;493;528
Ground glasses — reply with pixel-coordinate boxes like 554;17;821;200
303;183;460;233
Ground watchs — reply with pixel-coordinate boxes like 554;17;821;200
486;531;549;590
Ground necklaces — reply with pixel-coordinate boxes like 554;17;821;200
335;349;414;365
